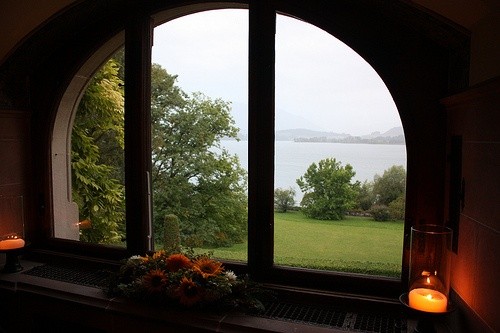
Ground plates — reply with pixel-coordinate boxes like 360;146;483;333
398;293;458;317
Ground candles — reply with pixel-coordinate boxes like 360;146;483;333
0;236;25;250
408;287;447;312
414;275;443;290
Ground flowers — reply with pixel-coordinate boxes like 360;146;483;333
100;249;278;313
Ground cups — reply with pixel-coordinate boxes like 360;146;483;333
1;195;24;250
408;224;450;314
